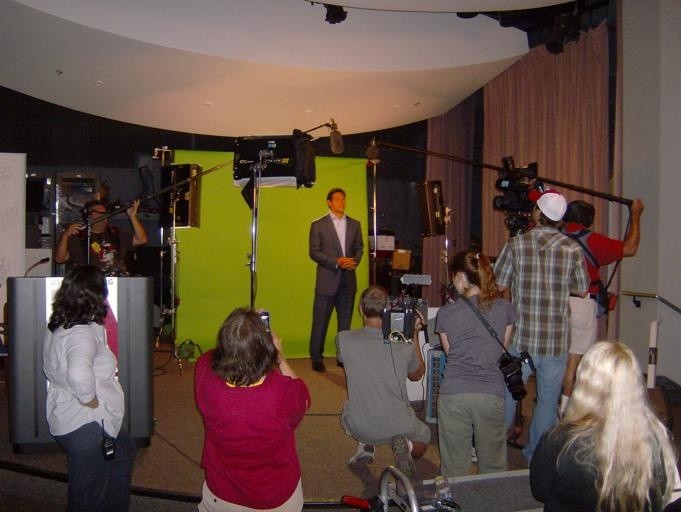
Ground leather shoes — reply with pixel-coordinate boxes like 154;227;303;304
311;361;327;373
337;360;343;368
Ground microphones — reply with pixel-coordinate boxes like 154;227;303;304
326;118;348;156
366;138;380;161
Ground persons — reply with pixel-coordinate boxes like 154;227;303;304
557;198;645;418
194;308;311;512
56;200;148;276
42;265;137;511
493;190;592;465
530;340;681;512
435;250;517;478
335;286;431;462
310;189;363;371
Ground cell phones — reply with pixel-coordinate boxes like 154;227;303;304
259;311;270;332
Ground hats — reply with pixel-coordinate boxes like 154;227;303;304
527;187;569;221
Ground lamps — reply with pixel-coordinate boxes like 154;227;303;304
324;4;347;26
545;13;582;54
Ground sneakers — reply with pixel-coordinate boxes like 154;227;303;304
387;433;419;481
346;440;376;472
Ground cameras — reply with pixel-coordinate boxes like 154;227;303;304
496;350;536;401
